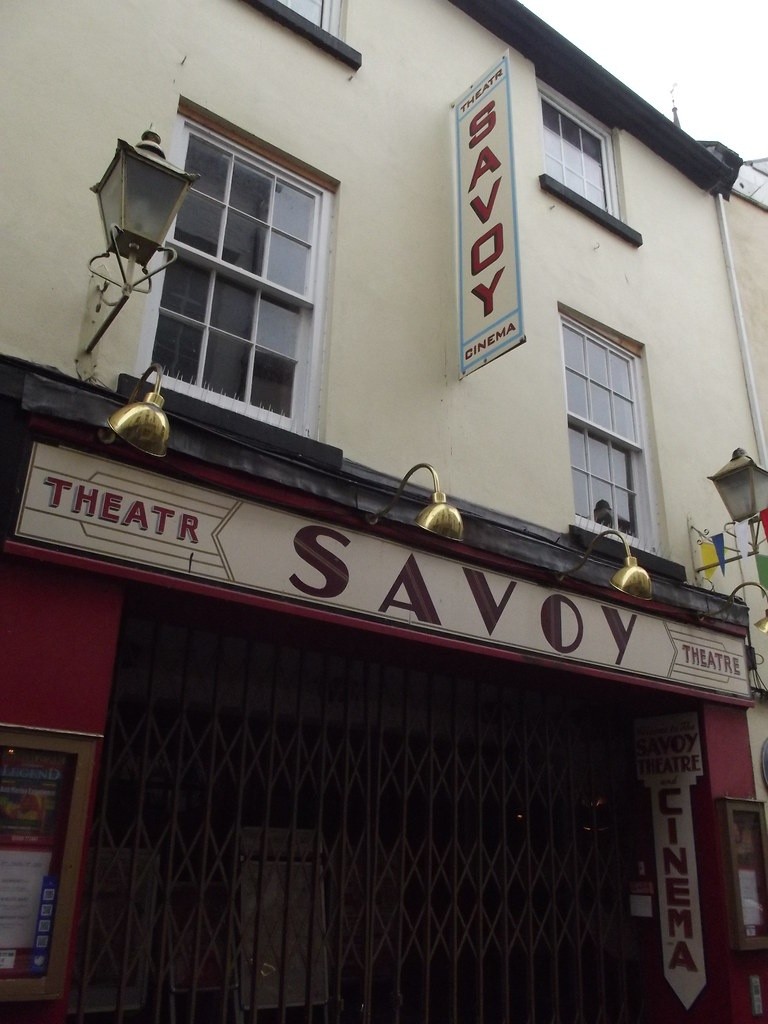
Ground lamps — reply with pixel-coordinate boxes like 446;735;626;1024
367;463;463;542
84;121;201;354
697;447;768;572
555;530;653;600
99;363;170;456
701;582;768;633
